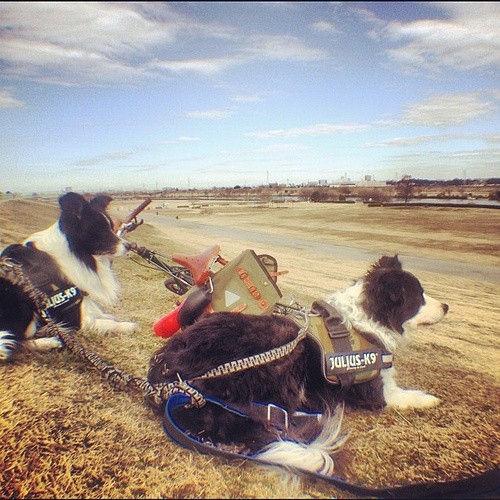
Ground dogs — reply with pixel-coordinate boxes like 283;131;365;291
146;252;449;478
0;190;141;362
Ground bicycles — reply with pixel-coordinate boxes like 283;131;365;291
108;198;310;319
